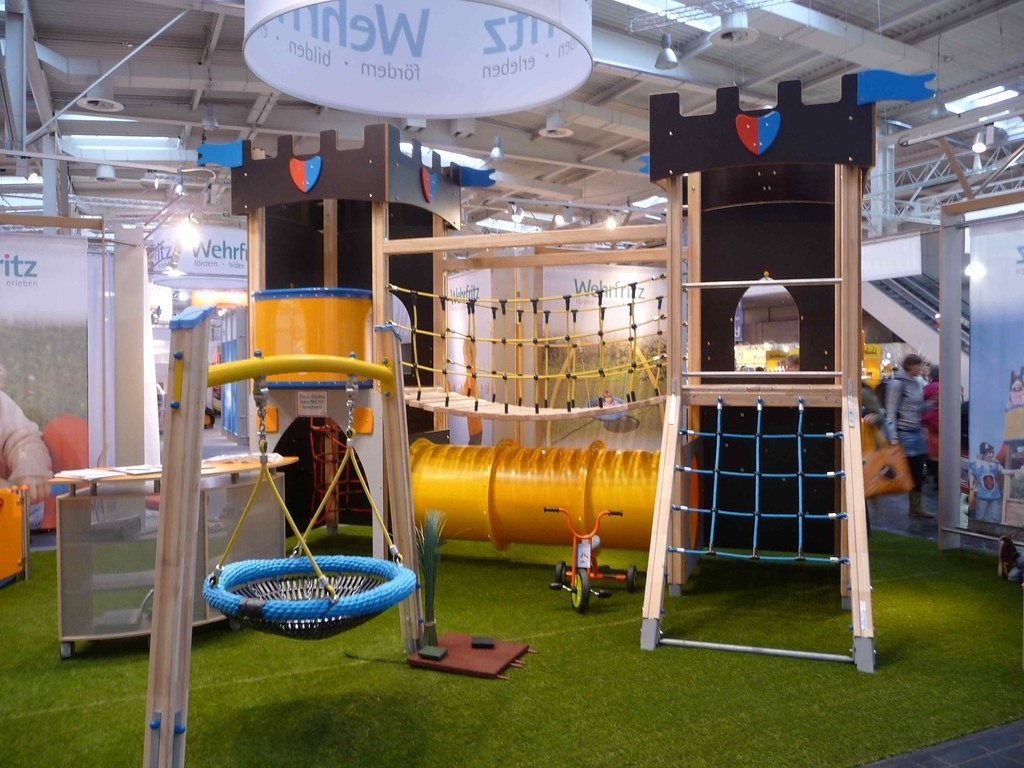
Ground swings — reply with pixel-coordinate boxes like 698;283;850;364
201;346;419;640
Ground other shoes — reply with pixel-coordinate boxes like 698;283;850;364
999;536;1019;564
997;540;1013;578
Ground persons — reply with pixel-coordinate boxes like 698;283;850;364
883;355;938;520
0;365;52;530
971;442;1019;523
787;355;799;370
604;391;620;406
1007;379;1024;408
862;386;884;429
924;364;939;491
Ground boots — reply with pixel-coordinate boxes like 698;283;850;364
906;488;935;520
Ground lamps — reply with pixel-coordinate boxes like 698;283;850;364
512;203;525;223
537;107;575;139
199;98;221;133
489;121;506;161
654;29;679;71
706;8;760;52
75;74;125;113
94;164;117;181
971;130;988;153
897;1;1024;147
555;206;574;225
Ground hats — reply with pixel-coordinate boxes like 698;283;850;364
980;441;995;454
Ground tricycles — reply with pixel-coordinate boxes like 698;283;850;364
544;506;637;613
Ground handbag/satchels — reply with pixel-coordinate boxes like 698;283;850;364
861;416;914;498
